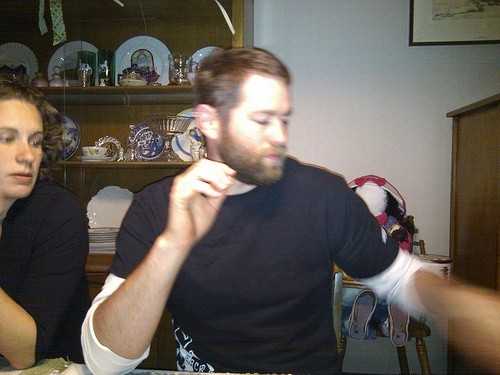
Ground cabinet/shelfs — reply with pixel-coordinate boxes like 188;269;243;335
0;1;244;369
446;91;500;375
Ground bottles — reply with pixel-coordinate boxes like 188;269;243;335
49;73;65;87
30;71;49;87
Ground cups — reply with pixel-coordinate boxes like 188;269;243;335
82;146;112;157
97;50;116;86
61;39;82;87
76;50;96;87
187;73;196;85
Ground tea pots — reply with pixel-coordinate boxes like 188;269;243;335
117;64;160;86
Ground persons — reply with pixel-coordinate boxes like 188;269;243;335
82;46;500;375
0;80;91;369
334;185;411;347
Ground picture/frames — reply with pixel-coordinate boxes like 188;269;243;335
409;0;500;46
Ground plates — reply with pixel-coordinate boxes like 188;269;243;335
109;36;176;86
184;46;226;72
88;228;120;254
47;41;105;87
0;42;39;85
74;156;113;160
127;122;166;161
86;185;134;227
171;107;208;161
50;111;81;161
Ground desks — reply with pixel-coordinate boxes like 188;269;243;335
334;216;451;375
